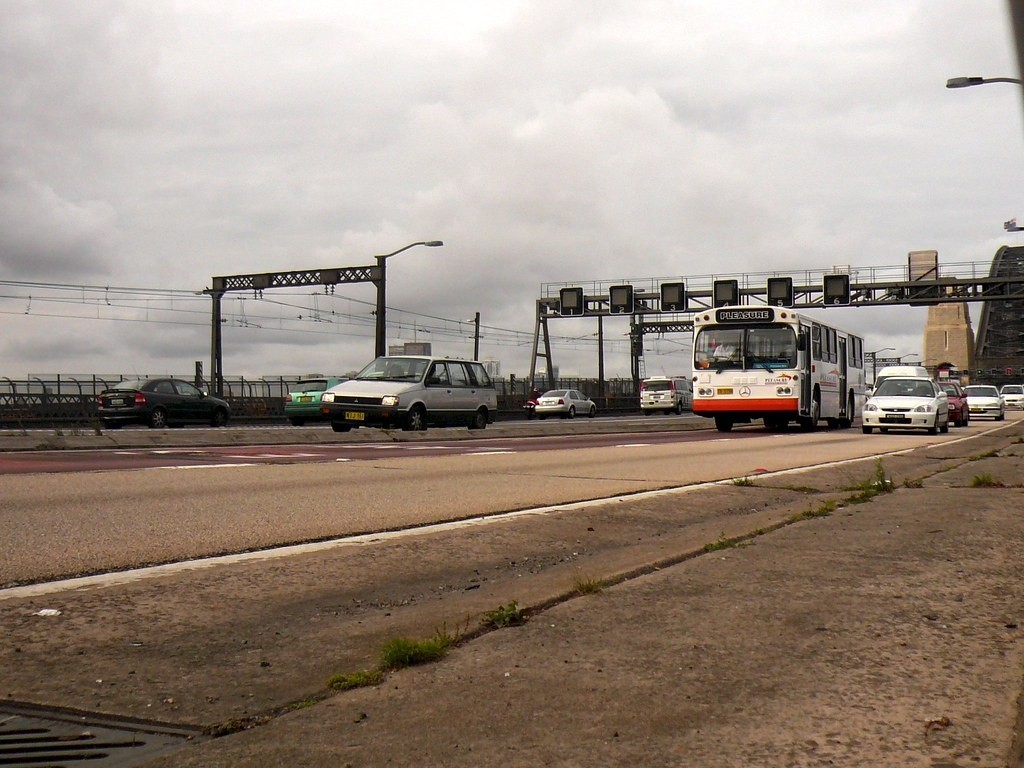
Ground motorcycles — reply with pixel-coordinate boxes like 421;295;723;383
522;401;535;420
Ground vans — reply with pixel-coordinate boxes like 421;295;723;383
865;366;930;399
640;378;693;416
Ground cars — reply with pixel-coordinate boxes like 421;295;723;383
861;376;949;435
320;355;497;432
937;382;969;427
534;389;597;420
964;385;1005;421
1000;385;1024;410
98;377;232;429
284;375;349;426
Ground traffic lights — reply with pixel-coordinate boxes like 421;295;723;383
990;369;997;375
964;370;969;375
1021;368;1024;375
1006;368;1013;375
976;369;983;376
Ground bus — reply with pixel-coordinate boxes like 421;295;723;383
693;306;865;432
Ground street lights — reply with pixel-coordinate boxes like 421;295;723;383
871;348;895;384
374;240;443;358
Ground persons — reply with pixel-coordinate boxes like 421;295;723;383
713;338;736;361
531;388;542;400
